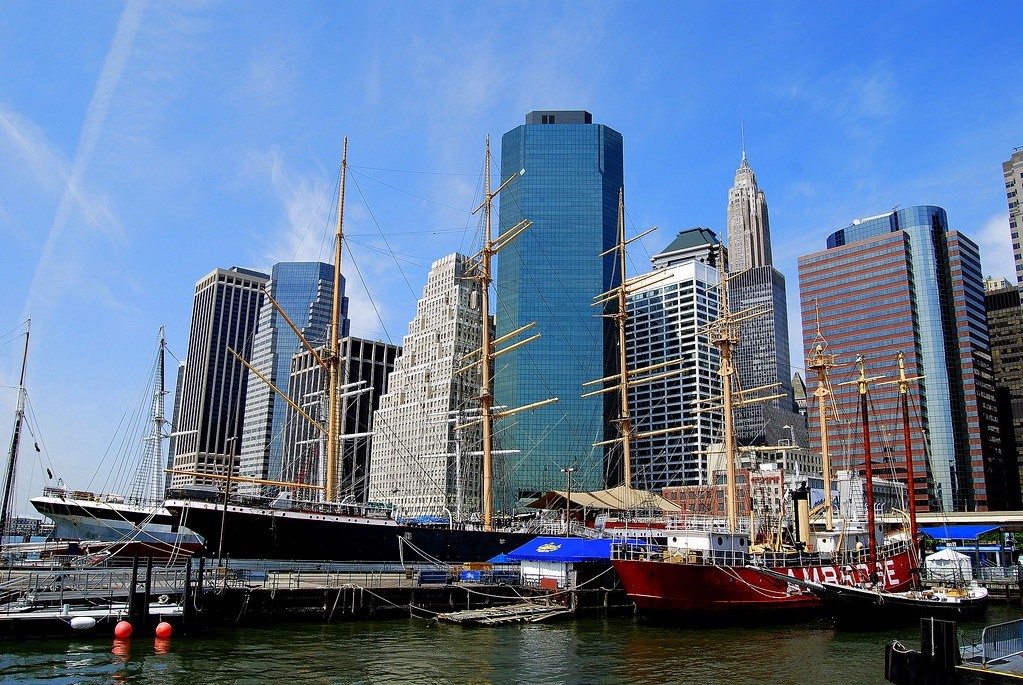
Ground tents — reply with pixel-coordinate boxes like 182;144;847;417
924;549;973;581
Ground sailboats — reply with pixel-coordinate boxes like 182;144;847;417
0;132;991;637
